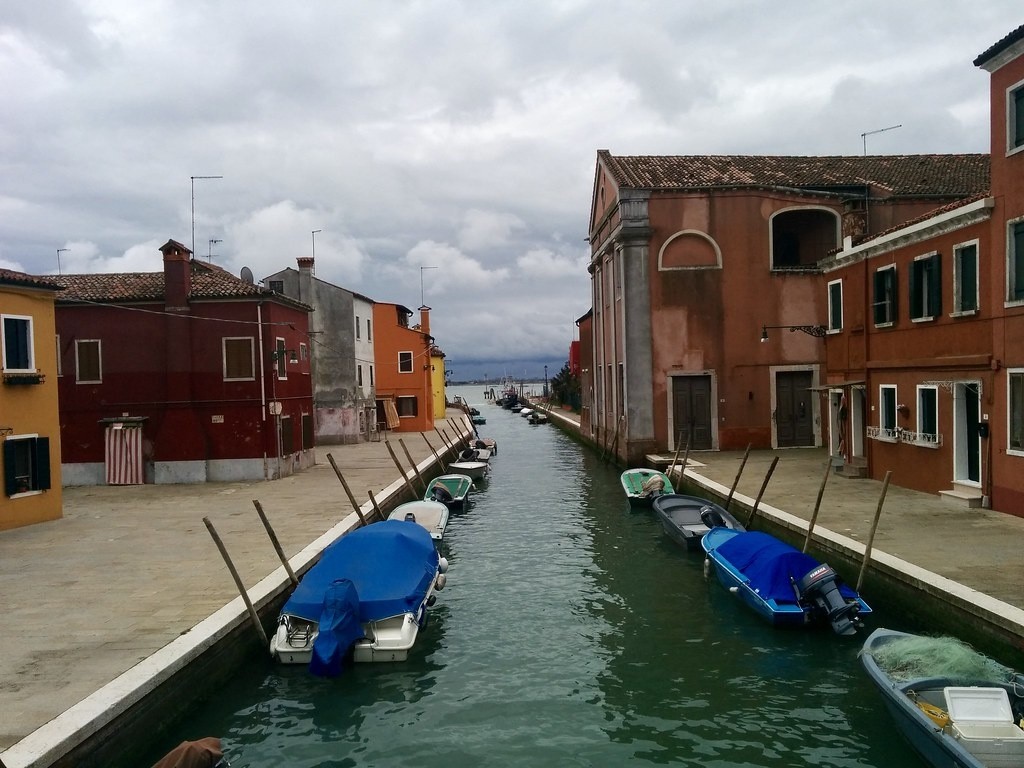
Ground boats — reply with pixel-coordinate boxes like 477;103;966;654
469;438;497;455
511;404;526;413
857;627;1024;768
469;407;480;416
653;494;747;552
460;449;491;463
472;416;486;425
520;407;535;417
269;518;440;663
424;474;473;511
449;462;487;481
386;500;449;540
621;468;675;509
527;413;547;424
701;526;873;627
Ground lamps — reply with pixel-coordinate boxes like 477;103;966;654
759;324;827;342
424;364;435;372
444;369;453;375
272;349;299;364
